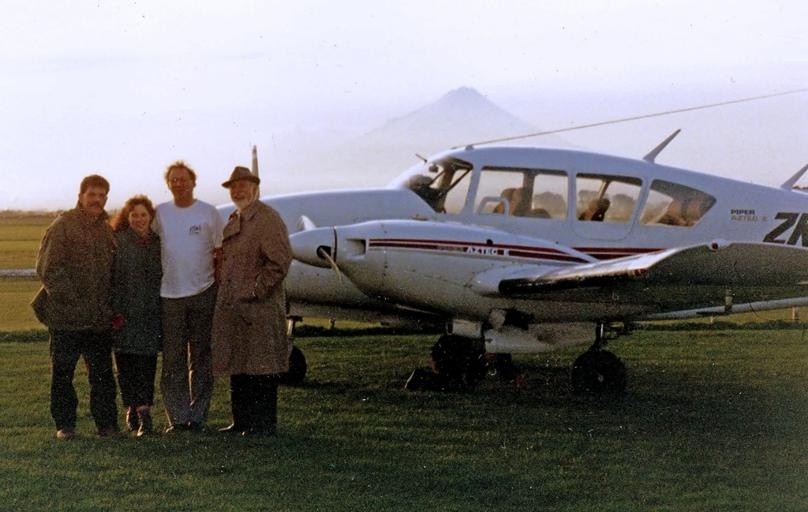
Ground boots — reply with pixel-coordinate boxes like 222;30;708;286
136;405;151;440
126;406;139;432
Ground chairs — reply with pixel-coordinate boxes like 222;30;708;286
578;198;612;221
492;188;534;217
657;193;712;228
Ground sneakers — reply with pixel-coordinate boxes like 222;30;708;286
57;423;74;441
219;424;276;437
96;426;127;438
166;424;189;434
189;421;209;435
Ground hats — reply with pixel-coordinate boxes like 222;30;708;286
222;167;259;188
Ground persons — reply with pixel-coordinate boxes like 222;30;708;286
31;174;127;439
212;166;294;436
152;162;226;434
110;195;164;436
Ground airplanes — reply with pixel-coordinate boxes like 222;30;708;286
1;129;808;398
